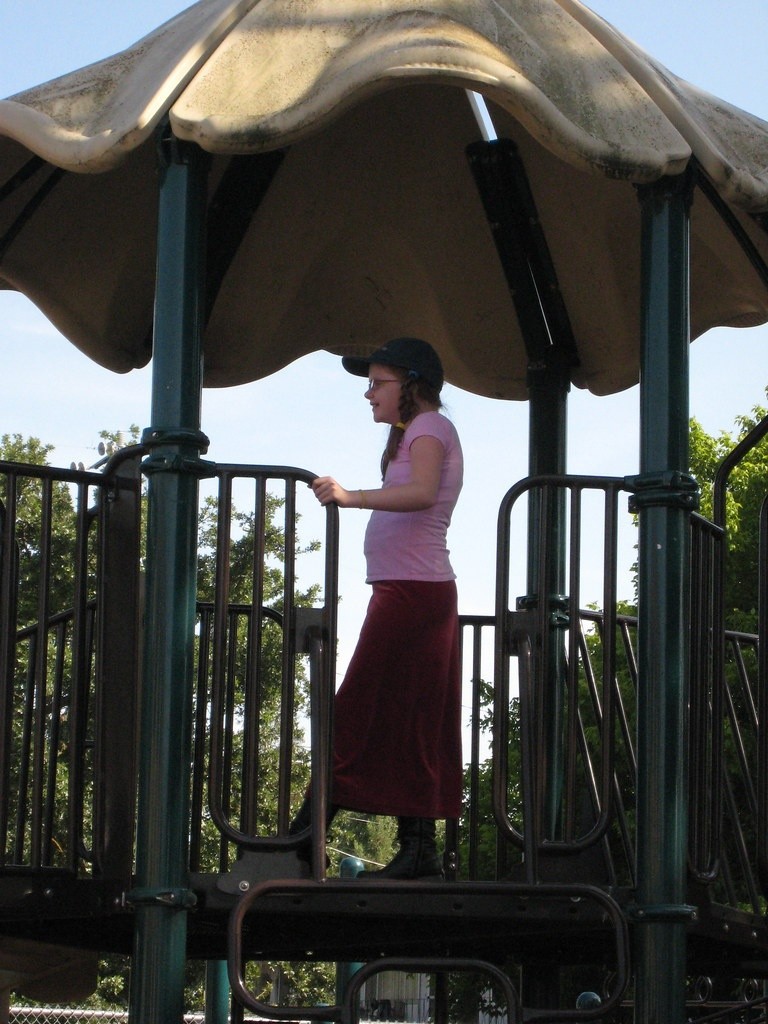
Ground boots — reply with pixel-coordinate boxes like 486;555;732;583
289;798;338;870
356;817;445;884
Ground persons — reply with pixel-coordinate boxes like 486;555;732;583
288;337;465;881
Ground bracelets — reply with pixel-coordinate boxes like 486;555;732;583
359;489;365;510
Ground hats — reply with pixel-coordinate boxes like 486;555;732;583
342;337;444;392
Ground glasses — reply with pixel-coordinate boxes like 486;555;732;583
369;378;402;389
